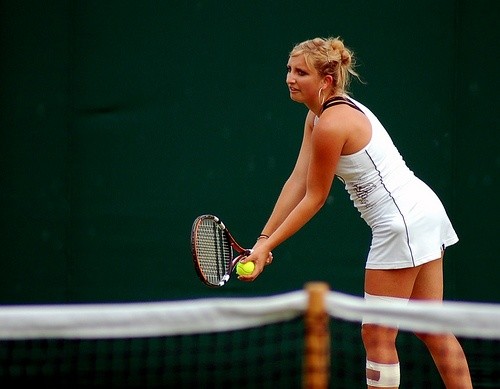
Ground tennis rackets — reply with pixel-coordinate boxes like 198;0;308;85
191;214;273;287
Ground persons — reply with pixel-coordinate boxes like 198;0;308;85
234;37;474;389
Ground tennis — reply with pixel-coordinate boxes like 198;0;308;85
236;260;255;276
